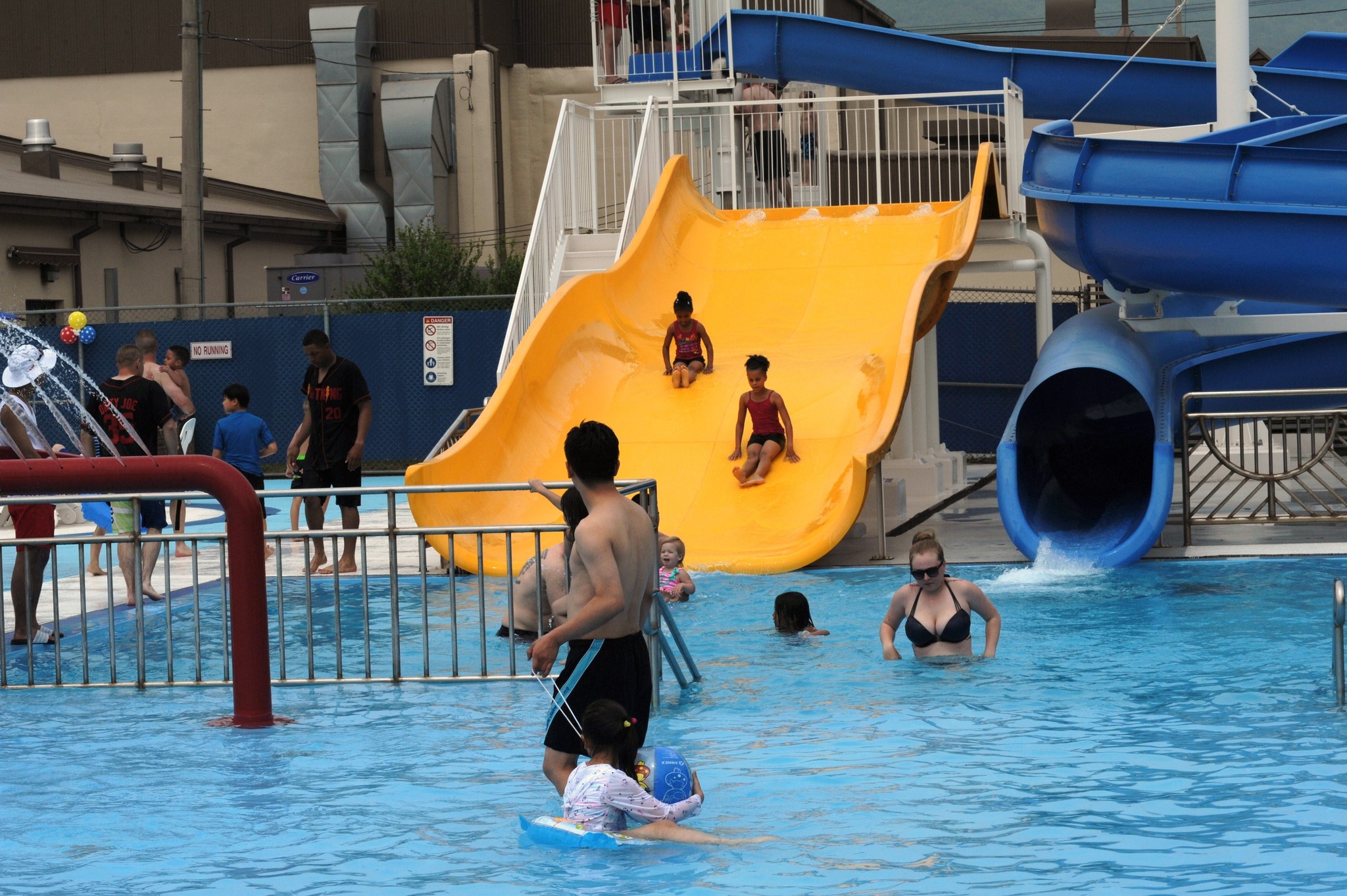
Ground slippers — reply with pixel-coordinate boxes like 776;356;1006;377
11;629;59;645
39;625;64;638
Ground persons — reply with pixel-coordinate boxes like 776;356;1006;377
742;74;794;208
527;478;588;665
598;0;691;84
794;91;818;186
662;291;714;388
285;398;332;541
527;420;658;798
287;329;372;575
0;344;65;643
879;528;1001;660
80;343;179;604
727;354;801;488
630;493;695;603
52;329;197;576
563;699;748;844
212;386;277;562
772;591;830;637
495;541;569;638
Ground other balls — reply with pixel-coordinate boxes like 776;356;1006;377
624;745;695;824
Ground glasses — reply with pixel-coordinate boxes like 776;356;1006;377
910;560;943;580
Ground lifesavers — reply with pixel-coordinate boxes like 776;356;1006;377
518;812;658;853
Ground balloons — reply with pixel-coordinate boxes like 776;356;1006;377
78;326;96;344
60;326;78;345
68;311;87;330
623;746;693;825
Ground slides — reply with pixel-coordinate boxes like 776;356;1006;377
402;141;1008;575
700;7;1347;575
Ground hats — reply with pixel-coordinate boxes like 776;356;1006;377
2;343;57;387
